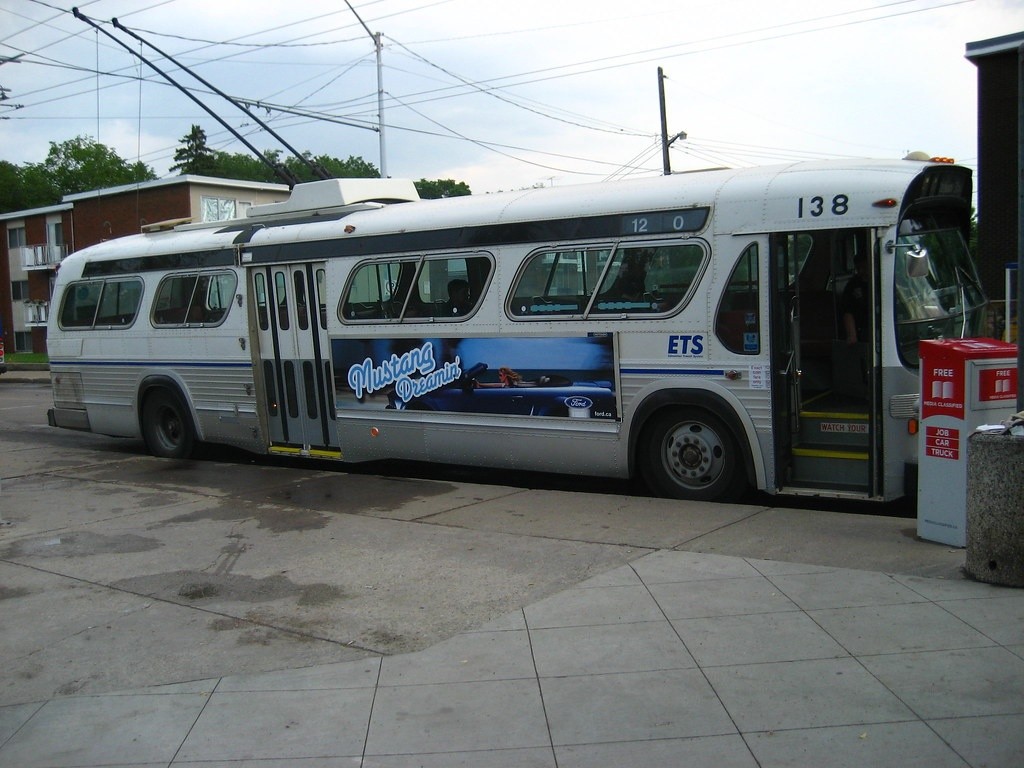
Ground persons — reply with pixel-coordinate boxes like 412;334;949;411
842;252;873;345
440;279;472;317
477;368;517;389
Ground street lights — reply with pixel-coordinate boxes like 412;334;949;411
657;66;687;175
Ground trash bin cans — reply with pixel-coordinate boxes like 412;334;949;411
967;410;1024;589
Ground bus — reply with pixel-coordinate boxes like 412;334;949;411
46;8;989;505
